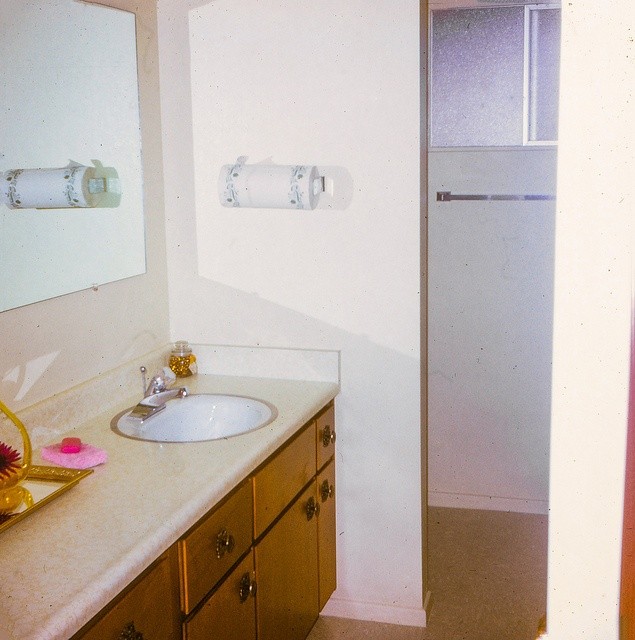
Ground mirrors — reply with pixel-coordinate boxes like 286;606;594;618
0;1;148;315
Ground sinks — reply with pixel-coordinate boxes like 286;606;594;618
140;396;273;442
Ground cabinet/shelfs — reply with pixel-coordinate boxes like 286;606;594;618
177;479;256;640
316;403;338;613
70;556;176;639
253;422;320;639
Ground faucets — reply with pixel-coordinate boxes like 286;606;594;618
139;375;189;409
